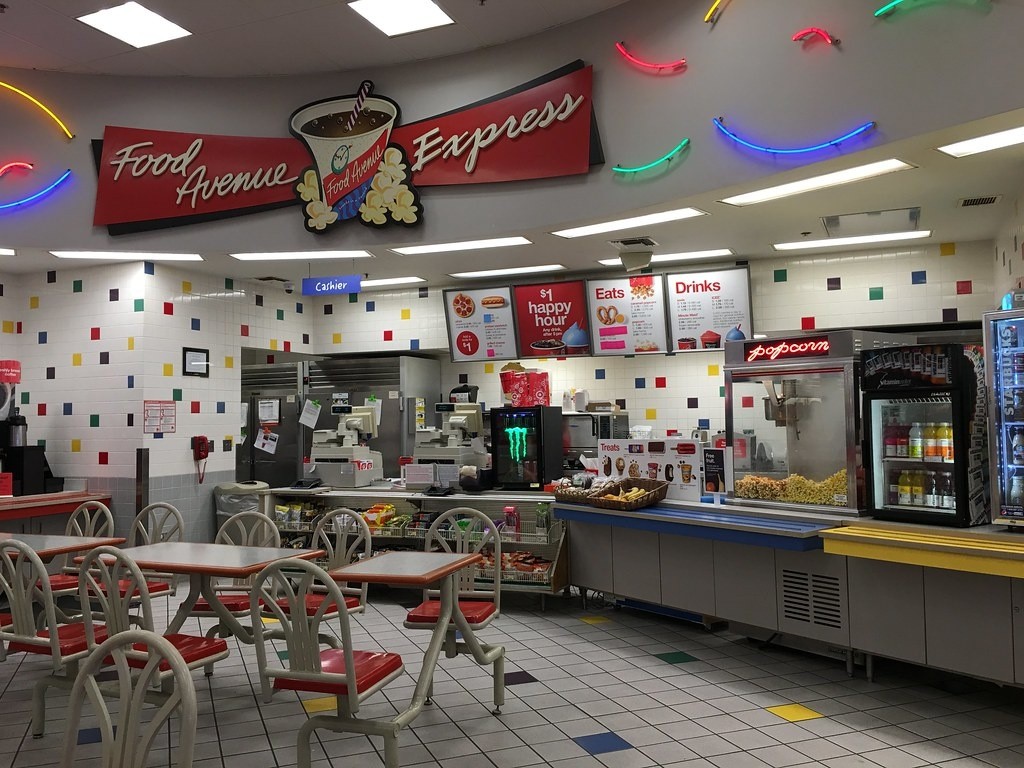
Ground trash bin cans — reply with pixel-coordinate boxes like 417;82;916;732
214;480;270;545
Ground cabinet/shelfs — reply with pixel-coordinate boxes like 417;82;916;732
270;512;569;611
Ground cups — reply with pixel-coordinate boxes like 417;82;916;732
682;466;691;483
530;340;566;356
701;336;720;348
566;344;589;354
678;340;696;350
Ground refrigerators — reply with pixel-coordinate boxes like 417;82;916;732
861;344;991;527
981;308;1024;530
491;406;563;490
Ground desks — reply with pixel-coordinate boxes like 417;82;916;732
69;541;327;702
0;491;112;538
0;533;127;623
327;553;505;735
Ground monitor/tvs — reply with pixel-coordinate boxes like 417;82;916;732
442;403;483;435
340;406;378;438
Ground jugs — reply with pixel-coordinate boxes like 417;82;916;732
9;415;27;447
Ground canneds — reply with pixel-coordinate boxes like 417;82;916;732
1013;352;1024;385
1013;389;1024;406
1005;326;1018;346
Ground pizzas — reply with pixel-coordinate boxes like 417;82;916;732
452;295;474;319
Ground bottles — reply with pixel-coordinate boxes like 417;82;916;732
884;422;953;459
1013;428;1024;465
1011;476;1024;506
888;469;956;509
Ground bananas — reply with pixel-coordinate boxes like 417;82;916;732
623;486;645;500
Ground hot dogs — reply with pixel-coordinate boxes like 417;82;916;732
482;296;504;307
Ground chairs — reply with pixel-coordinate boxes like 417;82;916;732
0;500;508;768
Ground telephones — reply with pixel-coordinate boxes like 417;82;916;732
194;435;209;461
